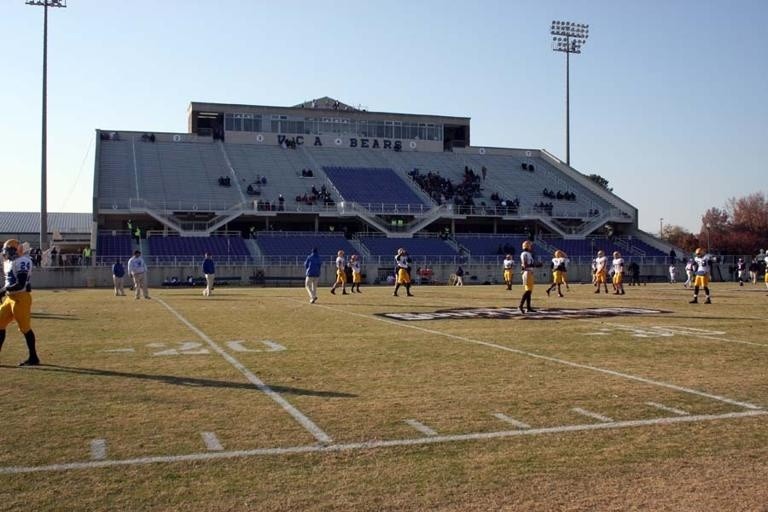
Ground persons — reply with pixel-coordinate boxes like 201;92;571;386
127;250;150;300
304;247;318;304
30;242;92;266
100;131;155;143
330;250;349;296
134;226;141;244
444;225;450;240
393;247;413;297
503;254;514;291
516;240;542;314
284;137;296;149
550;252;571;293
201;252;215;296
350;255;361;293
410;160;601;218
127;219;132;229
217;165;334;209
590;246;768;306
112;258;126;296
455;267;463;286
545;250;563;297
0;239;43;366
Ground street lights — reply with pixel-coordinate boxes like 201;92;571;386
549;17;592;169
659;217;665;240
25;0;71;256
706;223;712;254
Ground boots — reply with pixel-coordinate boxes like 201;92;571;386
18;329;40;367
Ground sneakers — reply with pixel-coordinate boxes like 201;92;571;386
704;297;711;304
134;295;152;299
545;286;570;297
593;283;641;295
526;307;536;313
670;279;758;289
392;293;399;297
202;291;211;296
115;292;126;296
330;290;336;295
506;287;512;290
517;306;525;315
310;296;317;303
357;290;362;293
342;292;350;295
689;297;698;303
406;293;414;297
351;289;354;293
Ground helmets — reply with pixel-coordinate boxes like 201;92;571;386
598;250;605;257
522;240;533;251
397;248;405;256
555;250;562;258
338;250;344;257
351;255;358;261
506;254;513;260
2;239;23;259
695;247;706;258
614;252;621;259
739;250;768;262
